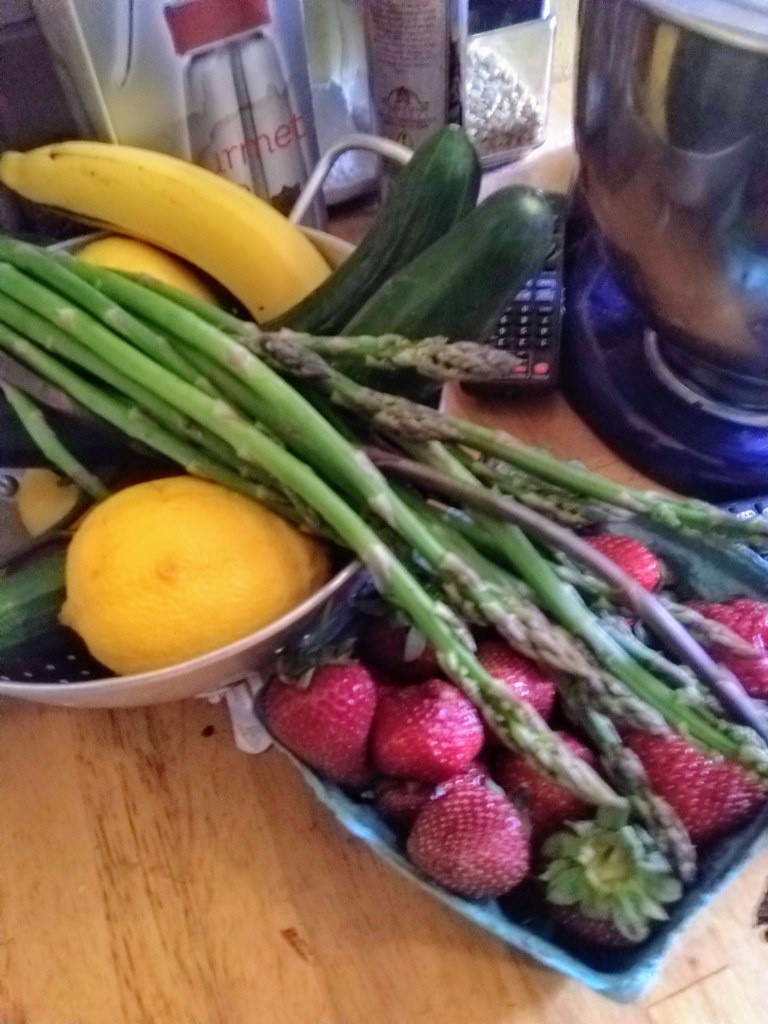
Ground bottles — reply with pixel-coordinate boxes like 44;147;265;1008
447;0;558;171
161;1;317;229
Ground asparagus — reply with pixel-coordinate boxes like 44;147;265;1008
1;237;766;871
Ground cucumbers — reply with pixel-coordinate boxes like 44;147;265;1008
3;121;555;660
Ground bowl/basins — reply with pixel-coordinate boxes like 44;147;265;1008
1;380;449;711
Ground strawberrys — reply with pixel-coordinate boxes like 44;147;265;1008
249;535;767;935
